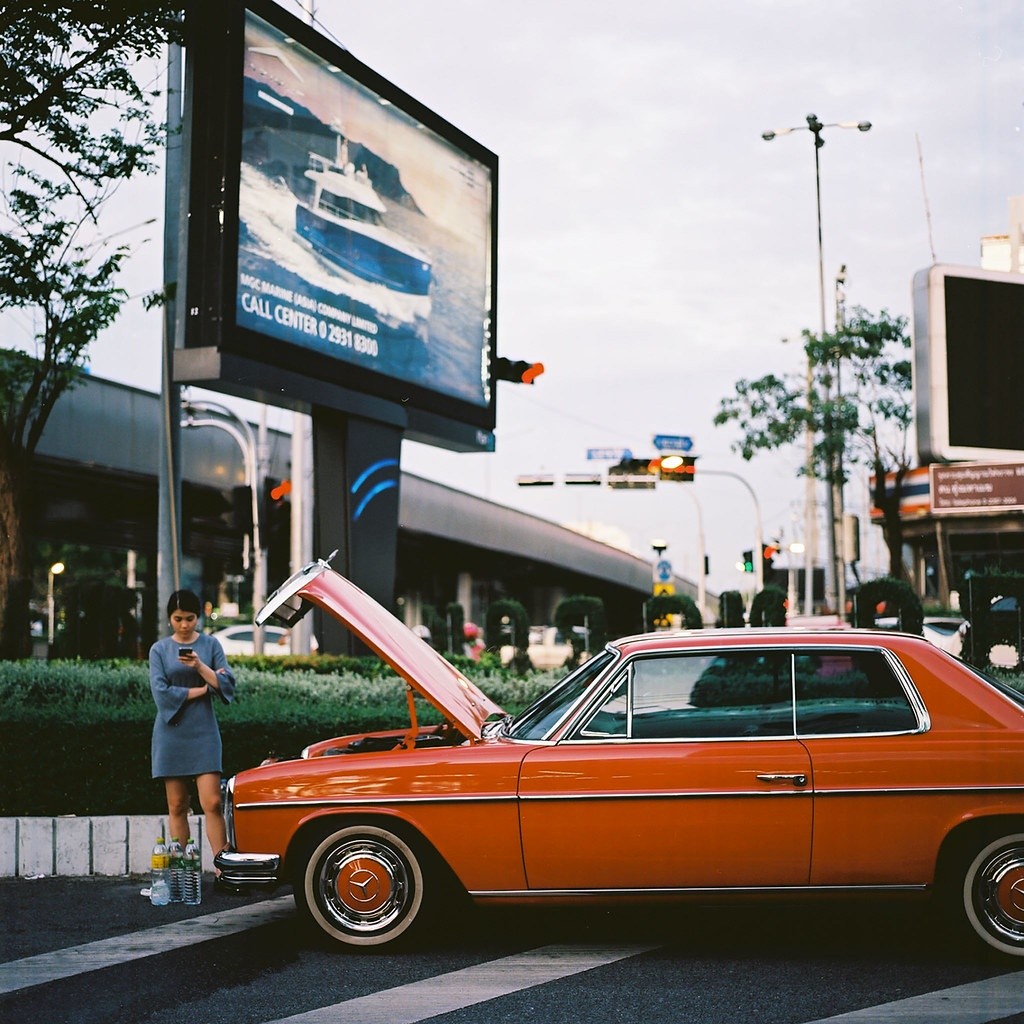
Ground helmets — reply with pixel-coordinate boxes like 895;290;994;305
464;622;479;640
411;625;431;638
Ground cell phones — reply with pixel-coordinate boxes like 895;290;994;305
178;647;193;657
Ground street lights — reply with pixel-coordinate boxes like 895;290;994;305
45;562;66;645
761;114;873;627
171;399;269;660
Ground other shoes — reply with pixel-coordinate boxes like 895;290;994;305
214;875;236;892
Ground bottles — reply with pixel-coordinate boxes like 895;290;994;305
151;837;170;906
184;839;202;906
168;837;185;903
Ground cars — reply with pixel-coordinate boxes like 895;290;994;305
205;623;294;660
214;552;1022;967
501;625;595;670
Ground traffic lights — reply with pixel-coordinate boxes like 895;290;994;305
496;355;544;386
618;457;653;473
761;543;775;586
650;454;697;483
259;476;295;557
229;483;254;537
742;551;754;574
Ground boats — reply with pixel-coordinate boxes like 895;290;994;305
299;131;436;331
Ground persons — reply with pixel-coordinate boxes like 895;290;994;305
148;588;236;884
